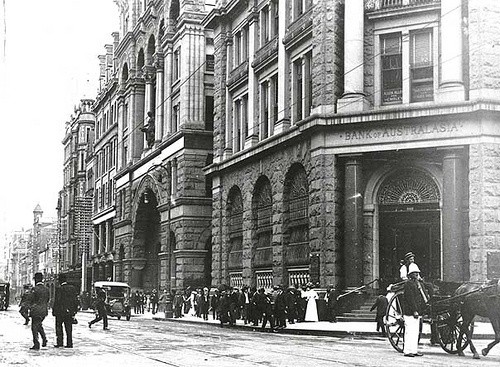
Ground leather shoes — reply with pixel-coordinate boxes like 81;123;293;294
413;352;423;356
88;322;91;328
30;346;39;349
64;346;73;348
54;345;63;348
404;353;415;357
42;340;47;347
103;328;110;330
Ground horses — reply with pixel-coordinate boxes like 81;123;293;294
450;279;500;360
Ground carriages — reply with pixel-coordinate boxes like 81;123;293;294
385;277;500;355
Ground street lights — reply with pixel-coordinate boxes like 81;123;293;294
144;164;173;317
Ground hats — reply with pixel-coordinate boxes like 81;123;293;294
56;274;70;280
386;284;395;292
403;251;415;263
408;262;421;275
33;273;43;279
100;285;109;289
24;286;30;289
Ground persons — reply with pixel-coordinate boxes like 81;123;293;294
52;274;78;348
386;284;396;326
402;262;423;356
18;285;30;324
399;251;423;280
29;272;50;349
370;290;388;335
80;283;338;329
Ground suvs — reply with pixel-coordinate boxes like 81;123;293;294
94;280;131;320
